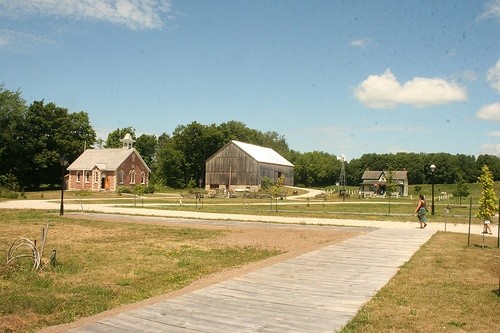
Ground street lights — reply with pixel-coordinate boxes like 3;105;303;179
430;164;437;215
60;153;70;216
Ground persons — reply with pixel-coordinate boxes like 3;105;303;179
482;220;493;235
415;194;427;228
